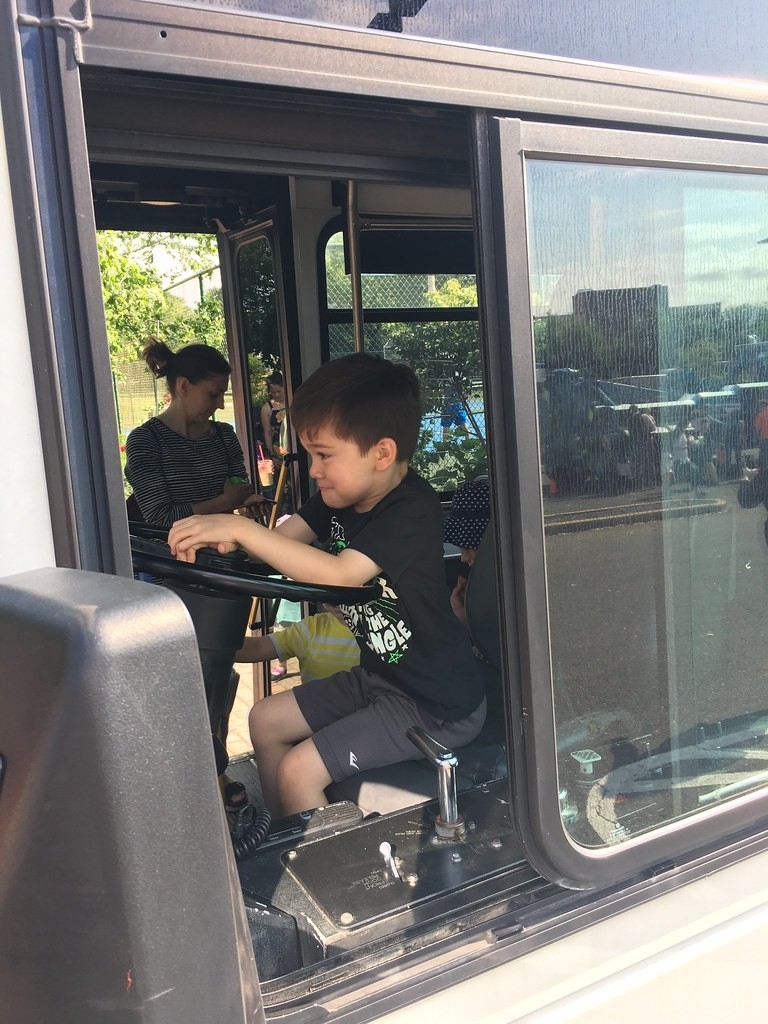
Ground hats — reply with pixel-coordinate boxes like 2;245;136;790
442;480;491;551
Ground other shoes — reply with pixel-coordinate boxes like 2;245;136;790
217;772;248;813
270;668;288;683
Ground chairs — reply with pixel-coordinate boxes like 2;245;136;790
297;510;535;821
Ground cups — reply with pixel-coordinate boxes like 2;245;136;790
257;459;273;487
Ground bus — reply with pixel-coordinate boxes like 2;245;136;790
0;0;768;1024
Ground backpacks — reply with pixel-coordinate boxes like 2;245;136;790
125;421;231;543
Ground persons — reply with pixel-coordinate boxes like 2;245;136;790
260;369;292;472
441;367;471;442
124;332;268;807
167;351;488;826
537;358;768;544
